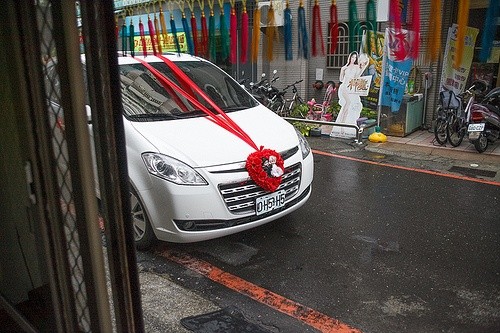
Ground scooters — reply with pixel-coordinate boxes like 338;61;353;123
467;87;500;153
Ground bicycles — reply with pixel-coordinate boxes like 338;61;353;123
433;84;477;147
269;78;306;118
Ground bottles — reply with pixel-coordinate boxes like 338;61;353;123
408;78;414;95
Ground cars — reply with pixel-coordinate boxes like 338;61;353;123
8;50;316;254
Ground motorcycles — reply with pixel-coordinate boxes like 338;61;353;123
237;69;279;107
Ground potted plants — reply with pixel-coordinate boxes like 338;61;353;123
290;99;378;136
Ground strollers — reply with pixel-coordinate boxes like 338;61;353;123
306;84;337;122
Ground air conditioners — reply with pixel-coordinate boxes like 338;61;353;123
255;0;286;28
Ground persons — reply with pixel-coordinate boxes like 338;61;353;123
330;50;369;138
262;155;284;178
